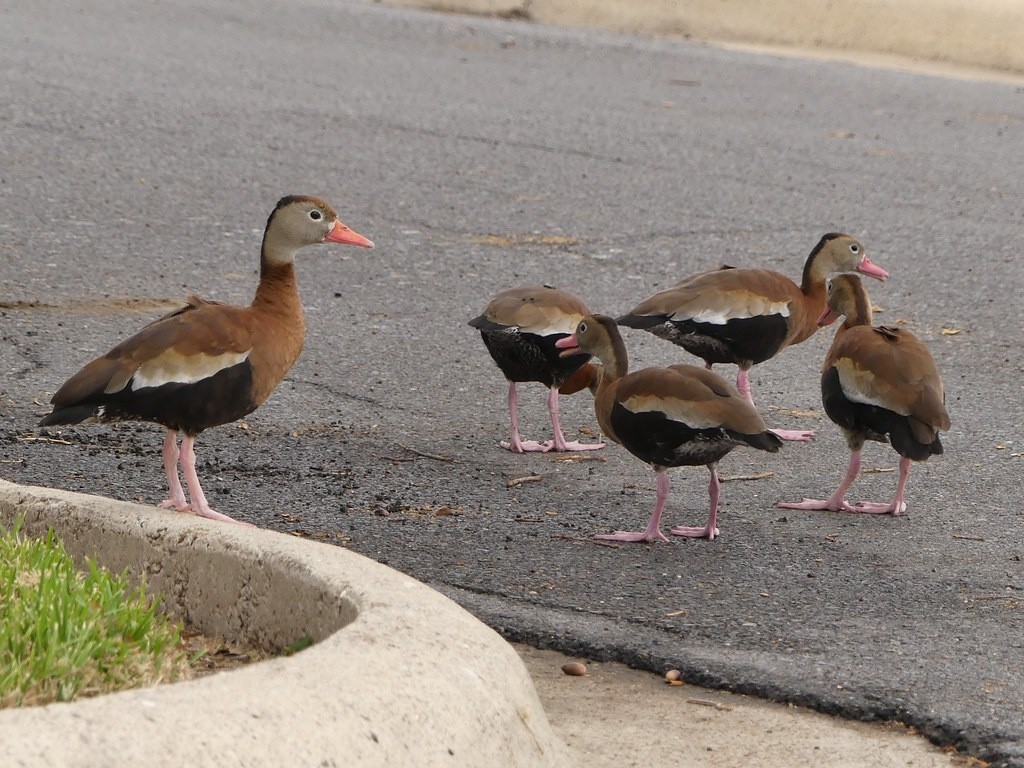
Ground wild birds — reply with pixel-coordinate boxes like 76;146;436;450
38;194;374;529
475;232;952;545
